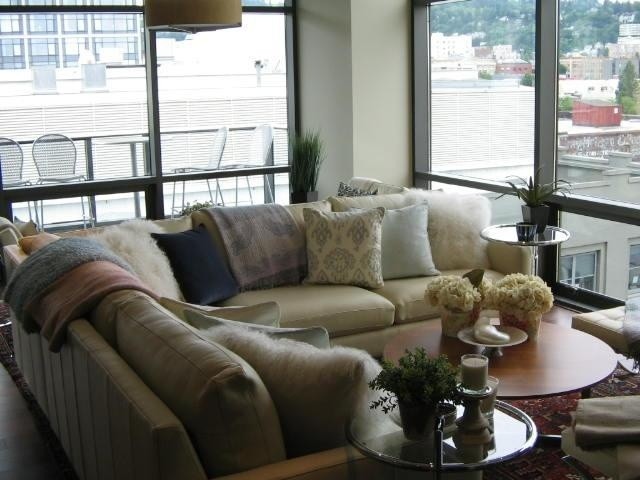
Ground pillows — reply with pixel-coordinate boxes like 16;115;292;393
157;294;389;455
148;223;241;305
301;204;388;290
10;215;40;237
398;186;493;270
326;195;443;283
87;217;181;302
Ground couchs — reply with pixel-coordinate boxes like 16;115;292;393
0;183;537;478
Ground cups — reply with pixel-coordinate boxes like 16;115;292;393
481;376;499;416
515;222;539;242
460;355;492;394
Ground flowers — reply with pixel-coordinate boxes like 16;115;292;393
486;272;554;320
424;267;495;313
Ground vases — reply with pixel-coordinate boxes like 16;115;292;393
498;308;543;339
439;308;481;338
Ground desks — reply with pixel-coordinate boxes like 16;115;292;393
478;223;571;275
95;133;178;216
345;396;537;480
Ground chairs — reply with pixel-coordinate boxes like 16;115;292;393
0;216;23;248
0;137;40;231
31;132;96;231
221;123;276;208
170;125;229;220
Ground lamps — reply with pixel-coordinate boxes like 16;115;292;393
143;0;243;35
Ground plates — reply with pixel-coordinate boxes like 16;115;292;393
457;324;528;348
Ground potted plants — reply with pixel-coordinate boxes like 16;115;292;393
289;127;326;204
494;166;573;234
366;342;463;438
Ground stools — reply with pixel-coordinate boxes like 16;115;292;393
559;398;639;479
570;305;640;399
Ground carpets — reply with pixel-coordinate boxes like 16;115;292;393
486;360;640;479
0;301;74;480
